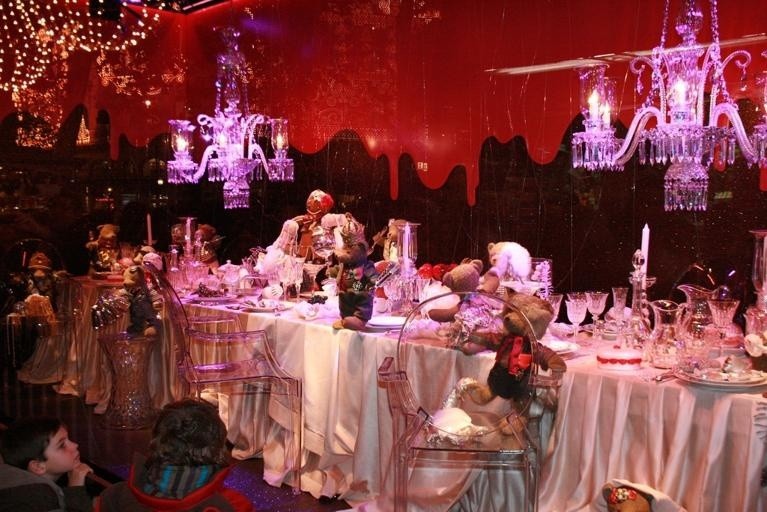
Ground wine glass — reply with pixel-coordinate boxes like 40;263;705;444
546;293;563;340
224;265;241;297
268;258;304;303
564;299;587;348
584;291;609;352
566;292;587;303
611;287;628;333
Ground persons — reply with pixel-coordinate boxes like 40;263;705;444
94;396;255;511
1;412;94;511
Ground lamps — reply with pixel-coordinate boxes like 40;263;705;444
167;23;294;211
572;0;767;209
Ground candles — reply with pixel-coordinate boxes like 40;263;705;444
403;221;410;272
641;222;650;290
147;213;151;245
186;216;191;249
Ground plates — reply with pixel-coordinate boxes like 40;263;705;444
671;367;766;389
190;293;236;301
549;341;580;354
242;299;293;312
586;328;631;340
364;315;407;329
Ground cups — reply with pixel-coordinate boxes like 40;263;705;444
384;279;430;319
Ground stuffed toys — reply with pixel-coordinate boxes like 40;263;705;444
464;294;567;436
193;224;221;273
86;224;121;267
121;264;160;336
481;242;512;293
326;242;379;329
373;220;409;263
290;190;345;263
428;260;510;355
8;253;67;312
168;224;187;257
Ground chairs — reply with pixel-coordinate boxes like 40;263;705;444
8;271;83;398
143;260;303;496
378;290;562;512
